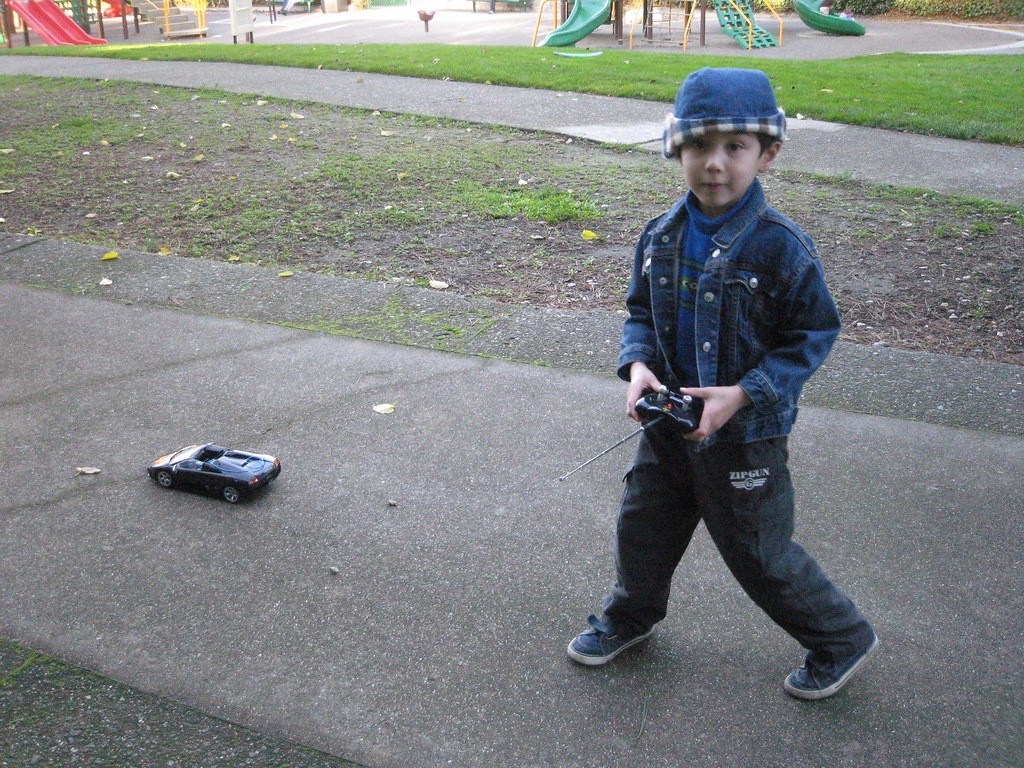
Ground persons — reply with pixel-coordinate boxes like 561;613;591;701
567;68;881;696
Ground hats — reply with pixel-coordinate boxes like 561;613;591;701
663;67;788;159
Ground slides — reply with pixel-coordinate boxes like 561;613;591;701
4;0;108;45
793;0;866;35
102;0;132;17
536;0;611;47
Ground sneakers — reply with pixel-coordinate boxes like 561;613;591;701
567;614;655;665
784;632;879;699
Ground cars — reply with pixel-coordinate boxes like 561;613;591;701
146;442;281;503
